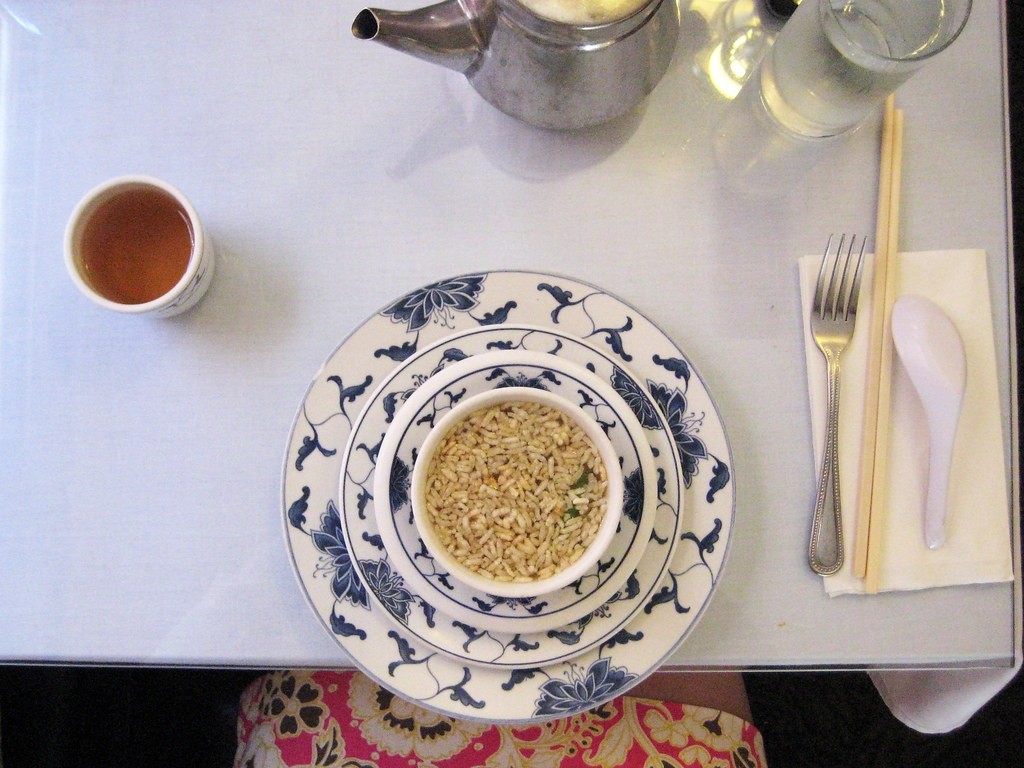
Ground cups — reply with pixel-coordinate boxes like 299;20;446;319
63;175;215;319
756;0;972;139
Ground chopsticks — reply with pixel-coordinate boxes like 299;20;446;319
849;93;905;596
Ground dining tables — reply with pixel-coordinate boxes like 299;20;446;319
0;0;1021;666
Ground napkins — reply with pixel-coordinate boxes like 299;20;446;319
799;247;1015;596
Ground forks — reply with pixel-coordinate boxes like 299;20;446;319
807;232;868;575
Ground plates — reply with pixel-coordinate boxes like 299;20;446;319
374;350;657;633
339;324;685;671
281;270;736;728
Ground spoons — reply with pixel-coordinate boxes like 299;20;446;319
891;295;968;549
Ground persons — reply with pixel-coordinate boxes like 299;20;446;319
234;665;768;768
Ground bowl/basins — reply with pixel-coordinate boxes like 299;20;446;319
410;388;624;598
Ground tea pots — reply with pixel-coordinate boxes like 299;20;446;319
351;0;682;133
